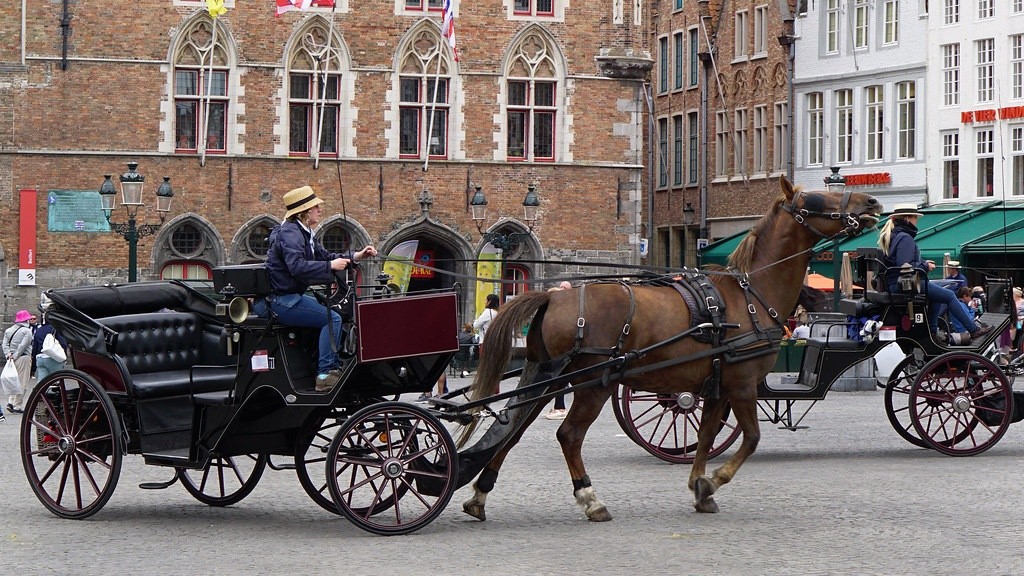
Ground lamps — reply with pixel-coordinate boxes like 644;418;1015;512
683;203;696;231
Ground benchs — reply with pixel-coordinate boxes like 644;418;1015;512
92;314;234;453
863;256;929;304
243;317;320;391
796;294;869;386
187;363;235;408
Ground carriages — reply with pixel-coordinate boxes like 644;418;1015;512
20;173;884;535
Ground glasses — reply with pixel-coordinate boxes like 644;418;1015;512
31;318;37;320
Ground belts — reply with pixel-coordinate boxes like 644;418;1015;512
12;353;27;356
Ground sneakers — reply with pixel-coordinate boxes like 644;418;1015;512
315;370;342;391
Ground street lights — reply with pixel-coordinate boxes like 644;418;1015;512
823;167;846;311
471;183;540;303
98;162;173;283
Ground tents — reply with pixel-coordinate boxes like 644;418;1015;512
695;206;1024;297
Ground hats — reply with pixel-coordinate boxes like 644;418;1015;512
14;310;31;322
972;286;983;292
282;186;324;219
1014;287;1023;296
945;261;962;268
889;204;924;216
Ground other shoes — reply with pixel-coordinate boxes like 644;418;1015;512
13;408;24;414
414;394;432;404
7;404;13;414
546;409;568;419
971;324;994;339
0;416;6;424
32;375;36;379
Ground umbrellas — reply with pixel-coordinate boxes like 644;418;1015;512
803;270;864;291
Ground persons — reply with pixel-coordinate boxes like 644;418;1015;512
783;321;811;339
253;186;377;391
515;310;532;339
876;203;995;340
32;311;68;383
28;314;42;380
453;294;501;396
560;281;572;291
413;392;432;403
0;310;32;415
538;287;566;419
942;260;1024;374
433;372;445;398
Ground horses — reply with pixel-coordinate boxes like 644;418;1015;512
454;169;884;523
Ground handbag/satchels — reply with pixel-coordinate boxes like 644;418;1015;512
1010;329;1016;340
314;277;354;322
1017;321;1022;329
41;330;67;366
0;358;23;395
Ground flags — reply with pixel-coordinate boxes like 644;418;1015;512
276;0;336;16
206;0;228;19
441;0;459;62
